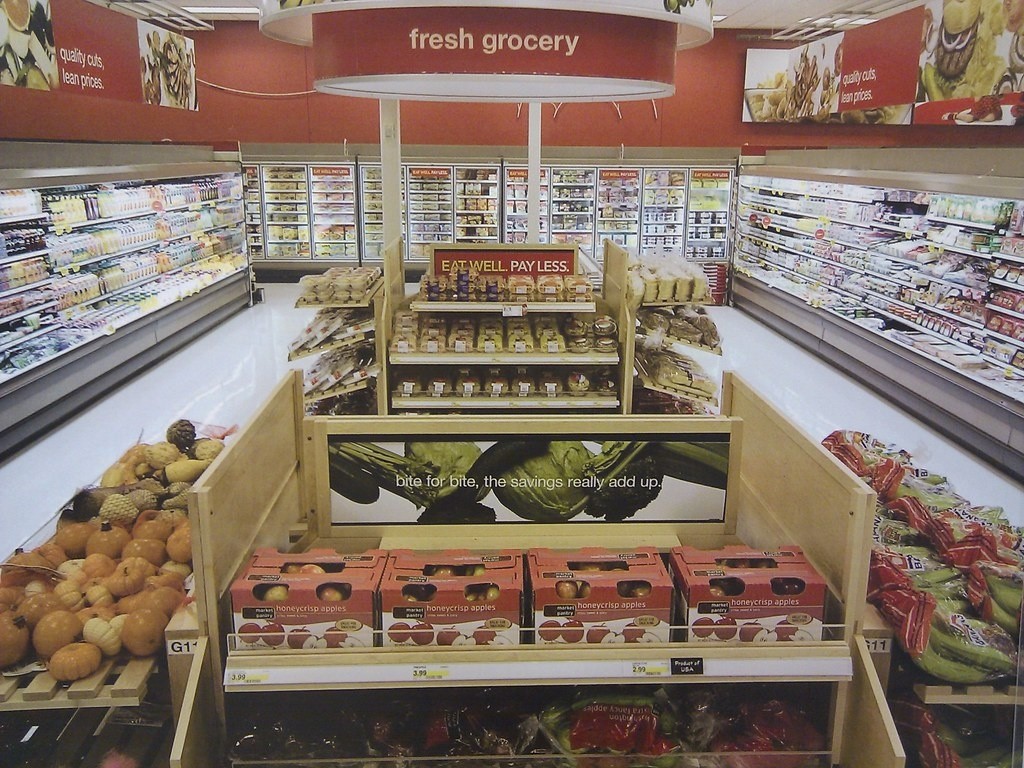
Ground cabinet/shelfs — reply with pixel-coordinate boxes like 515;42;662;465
287;235;724;419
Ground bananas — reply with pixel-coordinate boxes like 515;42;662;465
847;445;1024;768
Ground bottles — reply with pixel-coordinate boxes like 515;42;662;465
1;180;249;327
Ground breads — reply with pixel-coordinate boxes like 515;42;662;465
299;263;723;406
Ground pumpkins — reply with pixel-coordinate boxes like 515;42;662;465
0;420;230;682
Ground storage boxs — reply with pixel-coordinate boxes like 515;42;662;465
227;545;828;651
